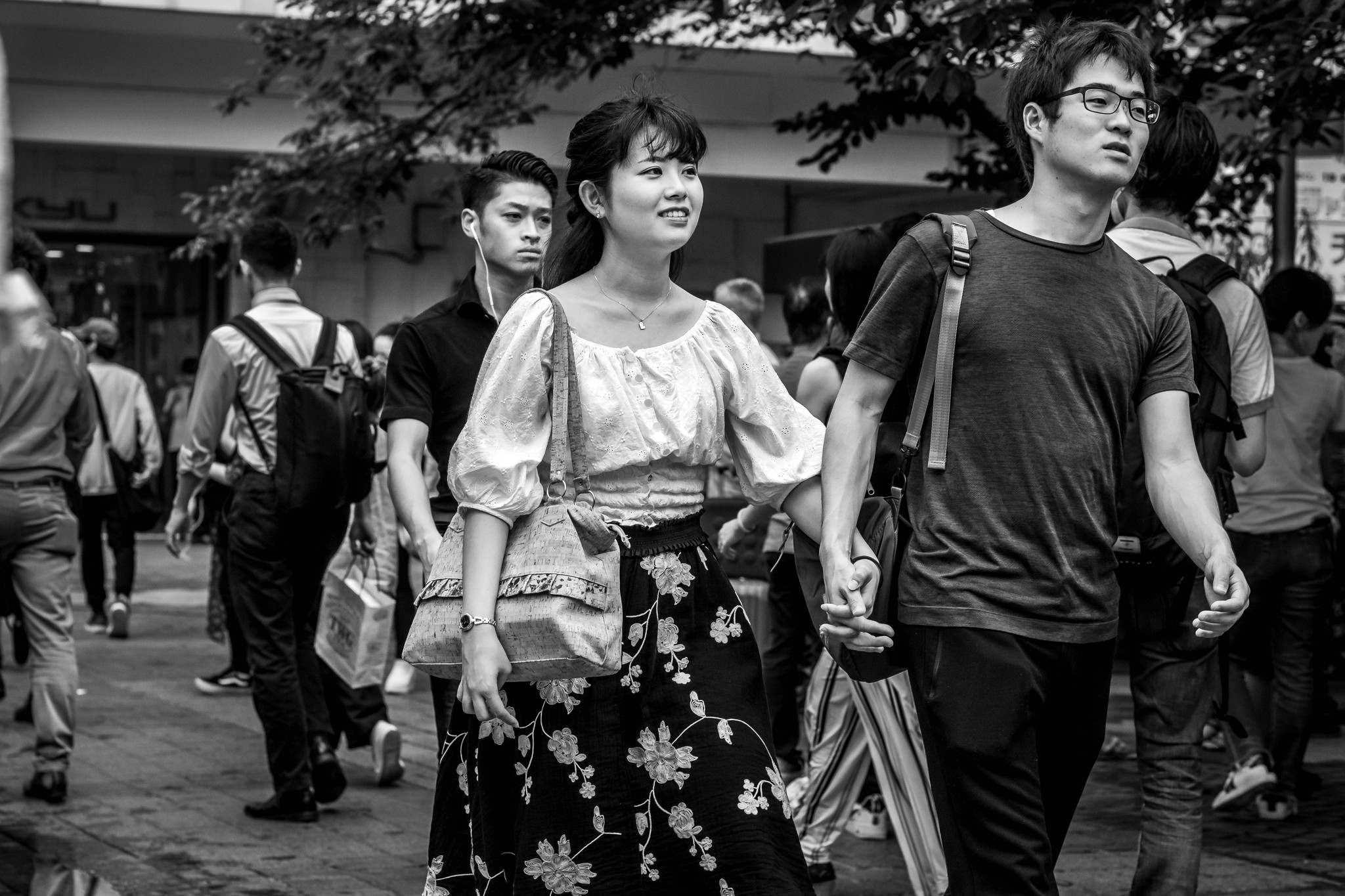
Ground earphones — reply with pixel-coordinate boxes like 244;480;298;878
469;222;479;240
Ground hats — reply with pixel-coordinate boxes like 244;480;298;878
67;317;118;347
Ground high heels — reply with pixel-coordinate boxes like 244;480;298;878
808;861;836;896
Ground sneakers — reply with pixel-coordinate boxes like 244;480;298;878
193;667;253;696
369;720;405;787
845;794;888;840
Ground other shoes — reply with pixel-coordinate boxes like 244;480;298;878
83;615;107;633
1212;753;1277;813
1098;736;1137;762
1202;725;1226;751
385;660;417;694
23;771;68;805
1255;791;1299;821
244;790;317;822
308;735;347;805
106;600;132;638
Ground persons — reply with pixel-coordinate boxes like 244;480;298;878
0;13;1344;896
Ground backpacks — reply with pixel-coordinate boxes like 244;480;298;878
226;313;376;515
769;492;910;683
1113;253;1240;568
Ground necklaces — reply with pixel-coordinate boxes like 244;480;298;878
592;263;672;330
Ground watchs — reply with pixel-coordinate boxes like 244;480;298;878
457;613;498;632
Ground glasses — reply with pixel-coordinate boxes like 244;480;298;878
1039;86;1160;125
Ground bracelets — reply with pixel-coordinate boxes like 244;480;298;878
736;508;755;536
851;555;885;590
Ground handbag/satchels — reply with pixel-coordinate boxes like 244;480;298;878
314;550;396;689
402;287;632;683
108;447;160;533
